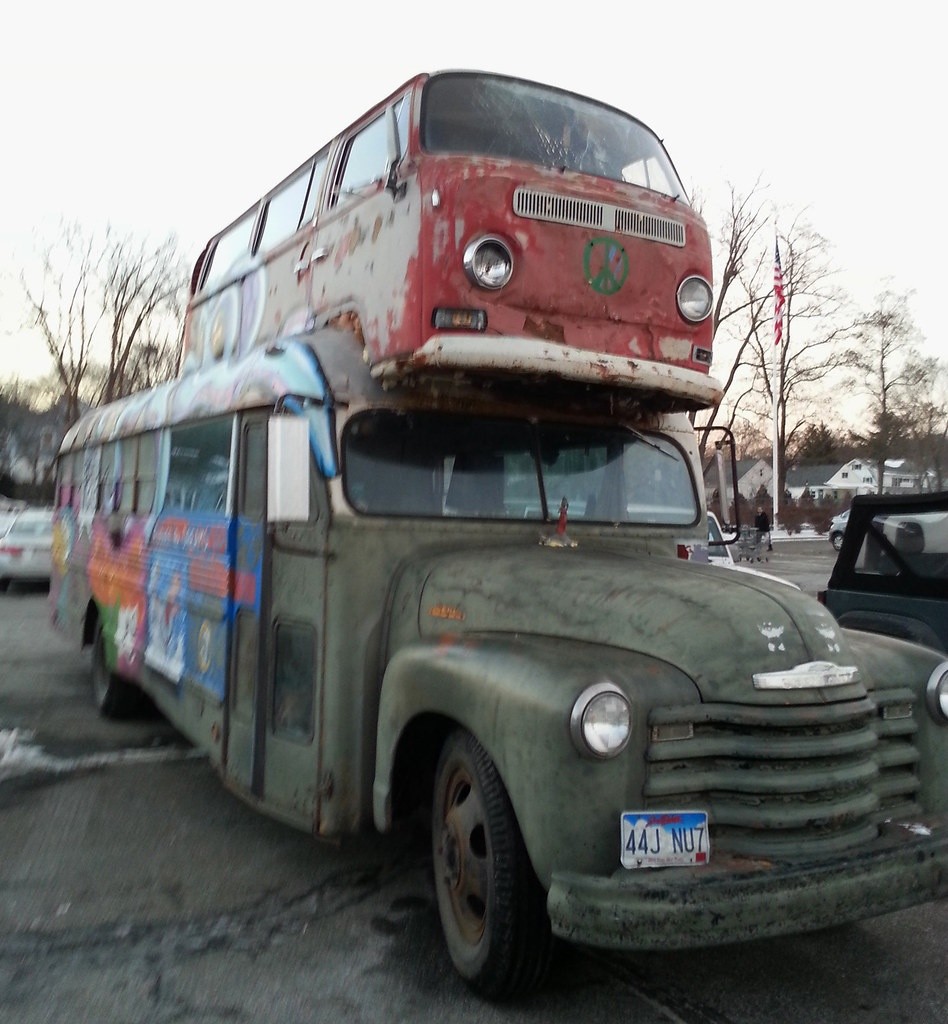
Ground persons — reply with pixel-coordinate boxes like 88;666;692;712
755;506;774;552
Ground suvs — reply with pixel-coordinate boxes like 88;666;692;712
522;492;948;650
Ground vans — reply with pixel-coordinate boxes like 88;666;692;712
179;68;729;409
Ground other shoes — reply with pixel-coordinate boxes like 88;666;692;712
767;547;773;551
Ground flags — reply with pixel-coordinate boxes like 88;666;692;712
773;239;785;346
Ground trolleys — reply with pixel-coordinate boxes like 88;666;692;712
732;528;762;562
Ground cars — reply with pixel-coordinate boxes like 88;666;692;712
0;502;54;590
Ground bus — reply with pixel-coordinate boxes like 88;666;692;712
49;337;948;1005
